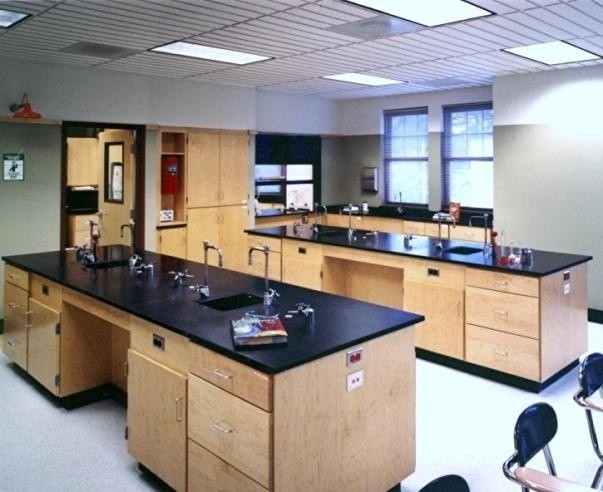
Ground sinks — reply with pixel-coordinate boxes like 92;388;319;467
444;246;483;255
321;230;347;237
199;292;264;311
85;260;129;270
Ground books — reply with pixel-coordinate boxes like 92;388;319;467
228;316;289;351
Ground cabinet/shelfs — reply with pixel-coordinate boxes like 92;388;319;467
361;217;491;242
68;212;100;248
185;205;250;275
280;236;346;297
463;254;587;384
155;127;186;228
125;312;187;492
246;229;281;282
255;210;361;229
27;271;111;399
185;127;250;208
4;261;28;373
186;323;415;492
403;255;463;362
158;226;186;260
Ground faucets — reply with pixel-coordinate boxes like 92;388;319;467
468;213;489;253
339;203;353;237
393;190;405;215
120;221;135;265
200;239;224;297
436;212;455;249
248;243;271;305
89;219;102;262
313;202;327;232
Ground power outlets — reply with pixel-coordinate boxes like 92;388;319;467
345;369;363;392
562;283;569;295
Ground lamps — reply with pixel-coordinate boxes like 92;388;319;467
8;92;41;119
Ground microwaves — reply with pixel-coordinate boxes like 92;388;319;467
67;185;98;214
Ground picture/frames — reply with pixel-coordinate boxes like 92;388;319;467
110;162;121;200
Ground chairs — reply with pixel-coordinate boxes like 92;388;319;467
417;474;469;492
572;352;602;490
502;402;599;491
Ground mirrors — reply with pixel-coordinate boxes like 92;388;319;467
103;140;124;204
